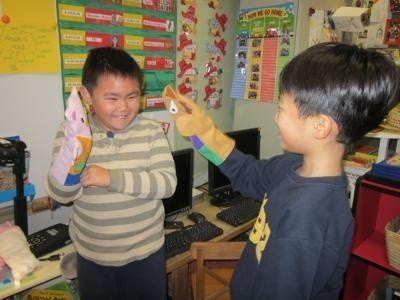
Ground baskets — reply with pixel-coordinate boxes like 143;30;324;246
368;274;400;300
387;103;399;128
384;212;400;269
372;152;400;181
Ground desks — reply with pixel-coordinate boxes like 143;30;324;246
0;186;254;300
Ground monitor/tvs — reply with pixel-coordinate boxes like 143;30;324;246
162;148;194;229
206;126;260;207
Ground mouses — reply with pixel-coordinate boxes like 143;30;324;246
188;212;206;224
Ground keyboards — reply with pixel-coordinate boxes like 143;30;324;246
165;219;224;261
216;199;264;227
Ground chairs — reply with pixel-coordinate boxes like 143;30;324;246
189;241;247;300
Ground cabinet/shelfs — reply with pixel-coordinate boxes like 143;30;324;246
343;154;400;300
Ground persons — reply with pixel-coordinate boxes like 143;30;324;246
111;35;122;49
237;51;246;68
162;39;400;299
45;45;178;300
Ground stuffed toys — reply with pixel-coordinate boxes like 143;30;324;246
0;219;43;286
